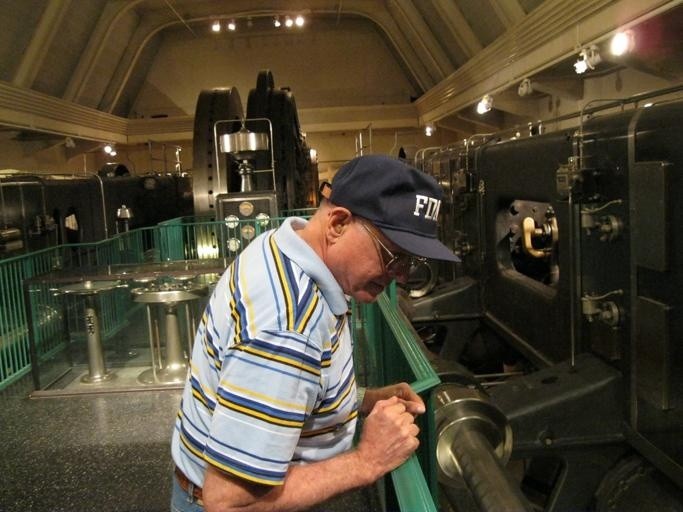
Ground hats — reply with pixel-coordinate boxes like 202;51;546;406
318;154;463;263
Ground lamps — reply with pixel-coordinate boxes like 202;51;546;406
213;15;307;32
477;93;491;115
104;143;120;160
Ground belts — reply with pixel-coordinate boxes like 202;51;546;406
174;465;202;500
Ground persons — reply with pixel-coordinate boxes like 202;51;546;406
165;155;464;510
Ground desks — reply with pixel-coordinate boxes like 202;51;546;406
25;258;229;390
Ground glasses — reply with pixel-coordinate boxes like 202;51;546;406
360;221;431;277
